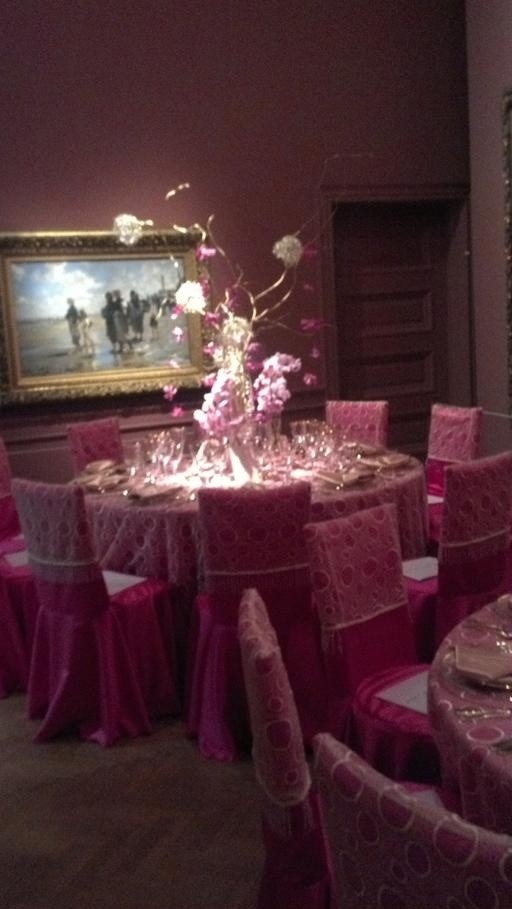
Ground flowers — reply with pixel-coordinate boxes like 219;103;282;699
112;178;330;436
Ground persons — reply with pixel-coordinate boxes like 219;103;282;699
64;289;163;356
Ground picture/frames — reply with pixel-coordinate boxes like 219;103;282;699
0;228;214;408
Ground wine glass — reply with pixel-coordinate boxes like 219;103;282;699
126;419;362;491
